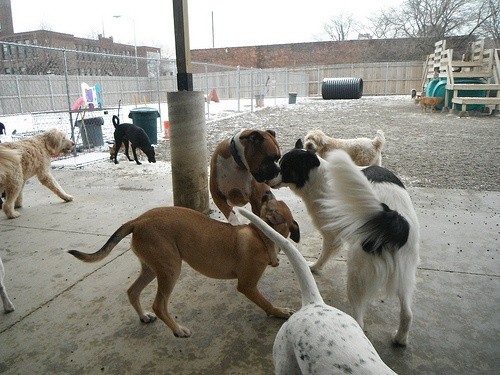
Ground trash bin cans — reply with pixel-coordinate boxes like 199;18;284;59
74;116;104;147
254;93;264;108
128;107;160;146
288;92;298;104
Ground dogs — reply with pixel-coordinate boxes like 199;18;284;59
66;190;301;338
303;129;385;167
236;207;399;375
0;128;74;217
414;96;443;114
109;115;157;165
209;129;281;227
270;138;420;346
